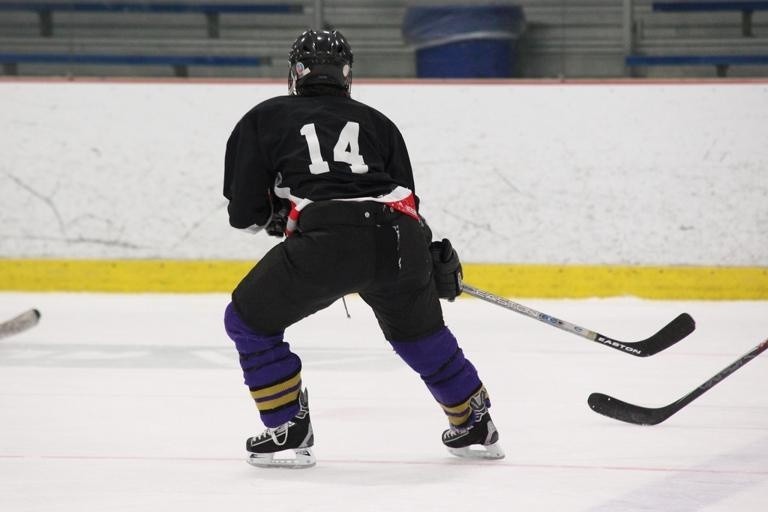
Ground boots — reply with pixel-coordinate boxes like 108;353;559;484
244;386;316;454
441;390;500;449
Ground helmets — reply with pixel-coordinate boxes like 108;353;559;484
287;30;354;91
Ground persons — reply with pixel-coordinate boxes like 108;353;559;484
220;28;499;454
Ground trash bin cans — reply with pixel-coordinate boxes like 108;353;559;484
402;6;526;79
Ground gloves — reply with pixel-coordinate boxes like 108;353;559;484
429;238;465;302
265;187;288;238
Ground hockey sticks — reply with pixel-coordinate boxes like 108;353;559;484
588;341;764;425
461;286;696;357
0;309;41;336
